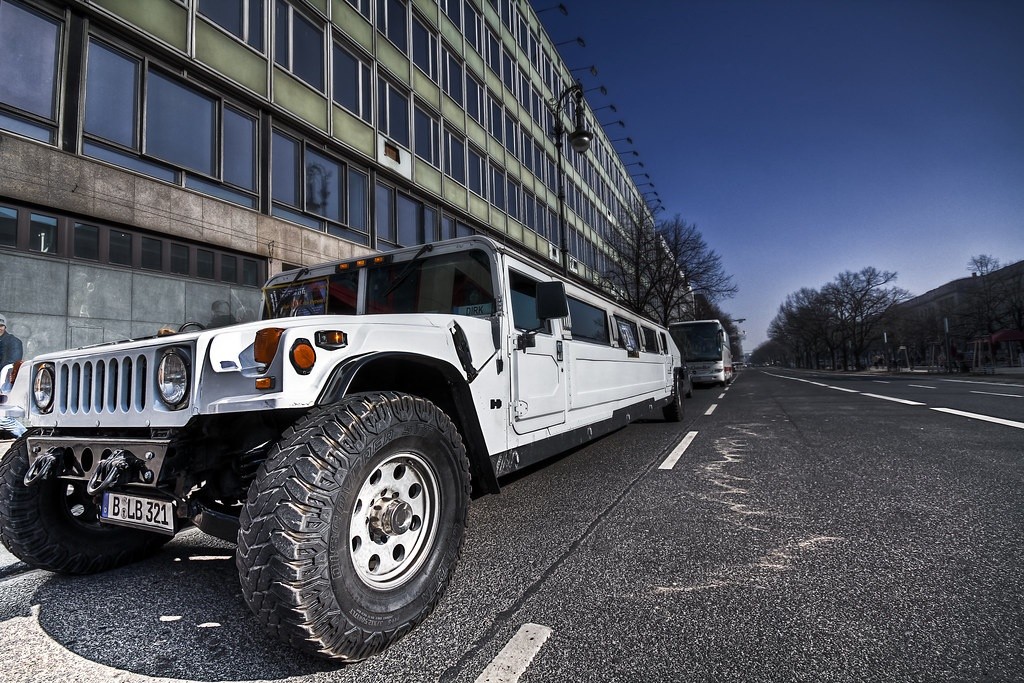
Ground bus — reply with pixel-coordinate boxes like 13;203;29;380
666;319;734;387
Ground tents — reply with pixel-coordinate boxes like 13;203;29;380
967;329;1024;367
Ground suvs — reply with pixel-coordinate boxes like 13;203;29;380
1;230;685;667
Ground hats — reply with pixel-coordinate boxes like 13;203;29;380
0;314;6;329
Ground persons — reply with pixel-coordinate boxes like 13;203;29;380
0;313;23;439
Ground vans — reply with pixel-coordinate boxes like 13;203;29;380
732;361;747;369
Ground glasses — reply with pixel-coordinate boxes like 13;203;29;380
0;324;5;327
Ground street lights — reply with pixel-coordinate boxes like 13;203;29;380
548;81;595;276
